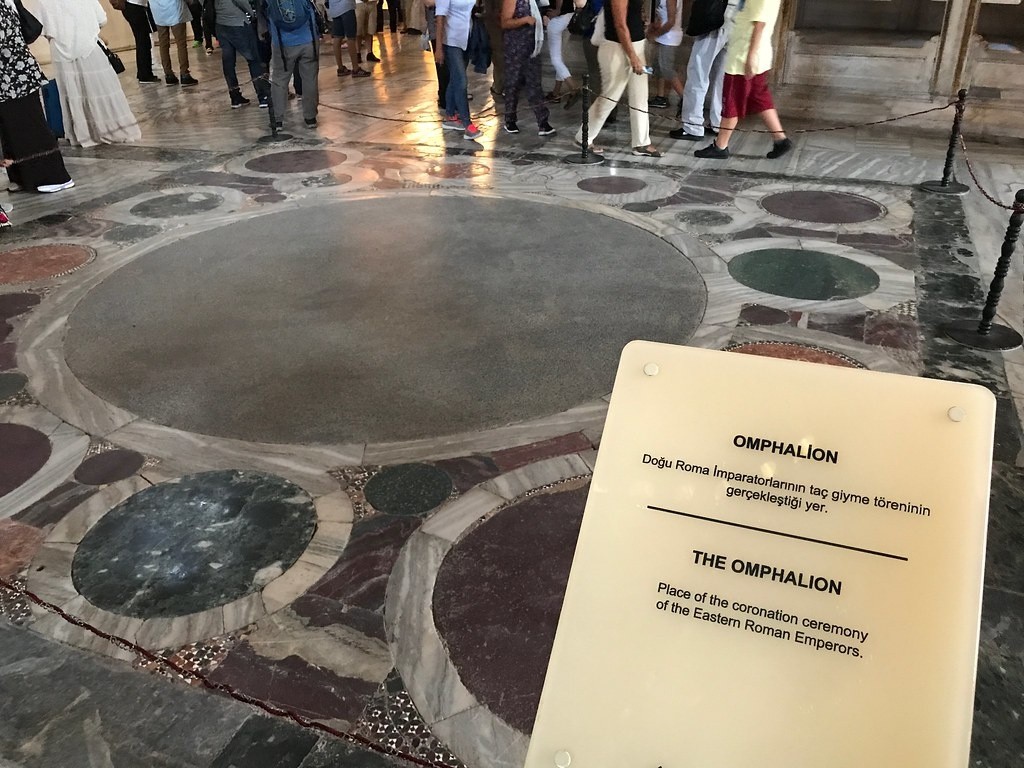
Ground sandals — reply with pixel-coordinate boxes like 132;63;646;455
632;145;666;157
573;140;605;152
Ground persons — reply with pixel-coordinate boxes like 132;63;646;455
0;0;792;216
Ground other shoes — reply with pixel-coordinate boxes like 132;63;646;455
192;40;202;48
339;52;380;77
275;121;283;131
206;47;213;54
257;96;270;108
287;92;295;100
297;94;302;101
304;117;318;128
0;205;13;228
215;40;220;47
232;96;250;108
37;178;74;193
166;76;179;85
137;72;162;84
180;73;198;86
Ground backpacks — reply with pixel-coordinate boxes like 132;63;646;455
108;0;127;10
270;0;312;32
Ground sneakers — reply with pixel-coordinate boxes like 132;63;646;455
438;84;583;140
649;95;795;159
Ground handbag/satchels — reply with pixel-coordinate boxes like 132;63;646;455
13;0;43;45
590;7;606;47
567;7;586;36
687;0;728;36
96;41;126;74
